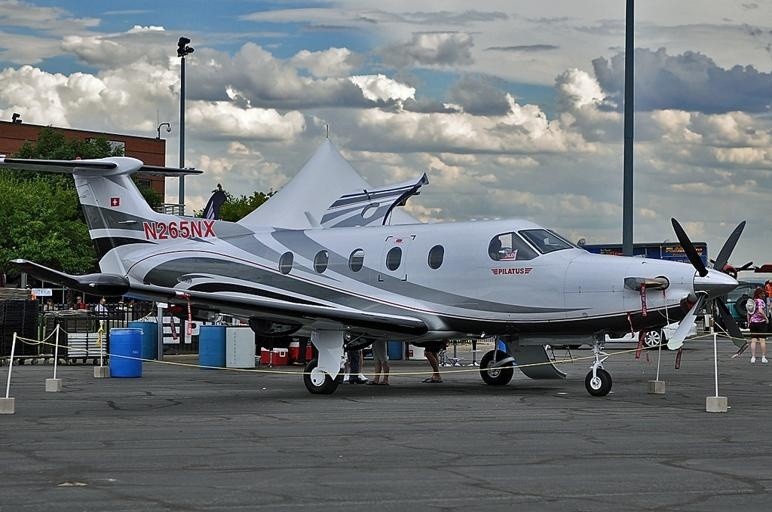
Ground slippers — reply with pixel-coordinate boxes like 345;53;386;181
421;377;444;385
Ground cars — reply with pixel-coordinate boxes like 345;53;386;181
604;319;698;349
45;309;97;351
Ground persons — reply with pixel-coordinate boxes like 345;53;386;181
347;347;369;384
746;287;769;364
755;279;772;341
419;340;443;383
366;338;391;386
490;239;507;261
25;284;136;316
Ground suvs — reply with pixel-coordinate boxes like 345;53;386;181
715;281;766;330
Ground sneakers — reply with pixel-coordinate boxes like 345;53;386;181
750;356;769;364
347;378;389;387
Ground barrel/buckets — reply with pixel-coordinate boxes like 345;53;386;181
495;338;507;353
109;327;142;378
388;340;402;359
199;325;226;368
128;321;155;360
155;323;163;359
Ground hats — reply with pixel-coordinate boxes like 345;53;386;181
746;298;757;314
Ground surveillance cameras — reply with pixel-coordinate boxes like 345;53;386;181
167;127;171;132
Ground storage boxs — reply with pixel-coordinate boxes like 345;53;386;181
260;340;312;365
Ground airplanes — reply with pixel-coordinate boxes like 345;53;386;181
0;156;748;396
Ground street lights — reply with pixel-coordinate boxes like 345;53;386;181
157;122;172;139
177;35;194;215
